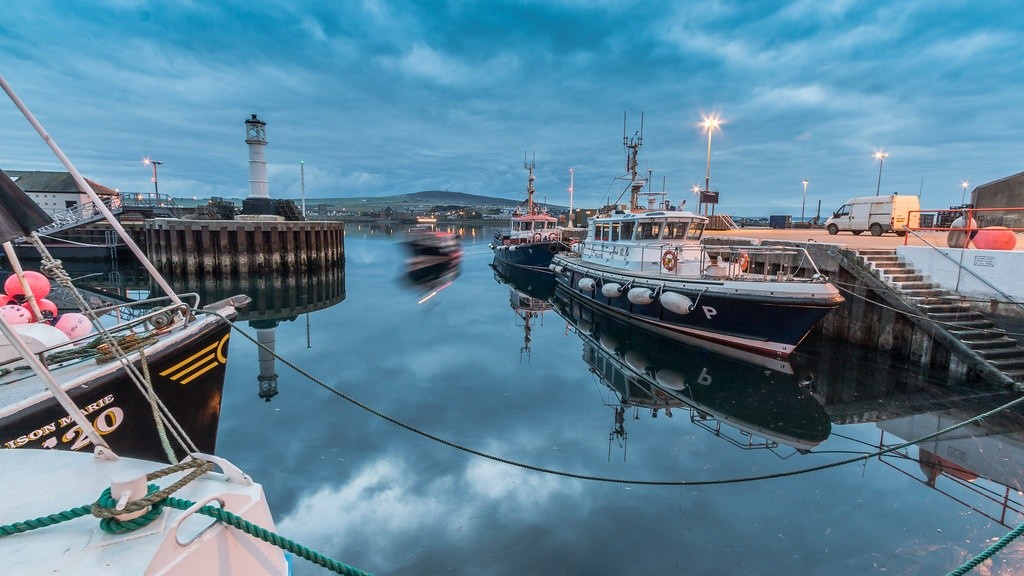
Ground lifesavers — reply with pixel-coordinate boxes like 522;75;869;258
737;251;749;272
662;249;678;272
548;243;559;255
548;232;559;241
569;239;581;252
531;233;543;243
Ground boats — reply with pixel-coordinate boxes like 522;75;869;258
488;254;557;364
548;285;832;464
548;111;845;361
394;222;462;259
0;78;291;576
487;148;578;272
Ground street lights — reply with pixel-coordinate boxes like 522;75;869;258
144;158;158;206
694;186;701;214
703;118;721;218
875;152;888;196
648;169;651;191
961;181;969;208
802;179;808;227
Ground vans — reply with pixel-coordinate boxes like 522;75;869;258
824;191;920;237
